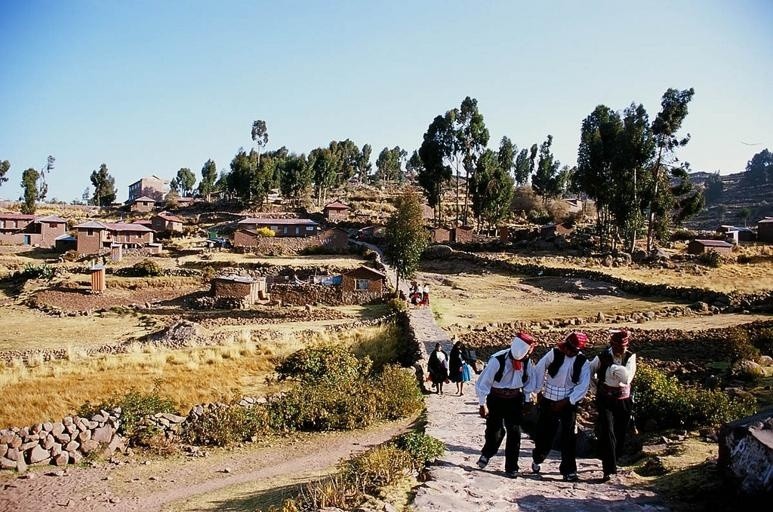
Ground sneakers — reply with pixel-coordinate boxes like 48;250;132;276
507;471;519;478
478;456;489;468
563;473;579;483
531;461;541;472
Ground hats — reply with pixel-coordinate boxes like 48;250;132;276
510;333;536;360
610;331;629;347
567;332;589;350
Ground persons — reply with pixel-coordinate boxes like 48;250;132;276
474;332;538;478
527;331;591;482
589;329;637;484
426;341;450;395
411;282;429;305
447;340;475;397
